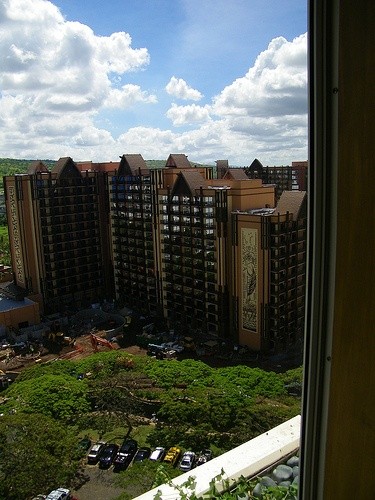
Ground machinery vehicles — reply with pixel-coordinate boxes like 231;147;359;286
89;333;120;354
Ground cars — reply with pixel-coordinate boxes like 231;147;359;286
88;441;107;463
195;448;213;467
163;447;180;468
132;447;152;465
150;447;165;461
45;488;70;500
180;451;195;471
100;444;118;469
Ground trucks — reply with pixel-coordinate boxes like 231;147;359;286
147;344;178;360
161;342;184;353
204;340;234;365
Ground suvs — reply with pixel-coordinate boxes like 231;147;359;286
115;440;137;469
